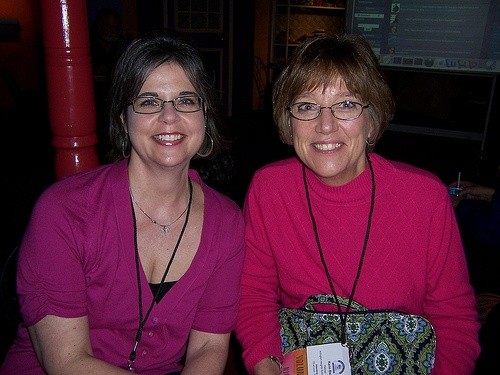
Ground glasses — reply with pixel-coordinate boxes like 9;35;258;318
284;100;371;121
125;93;203;114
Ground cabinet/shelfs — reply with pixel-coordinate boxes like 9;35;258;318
268;0;346;85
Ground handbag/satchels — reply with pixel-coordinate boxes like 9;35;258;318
278;293;437;375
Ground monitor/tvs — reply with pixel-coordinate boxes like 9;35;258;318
345;0;500;74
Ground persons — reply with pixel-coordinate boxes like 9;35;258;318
234;32;481;375
0;37;243;375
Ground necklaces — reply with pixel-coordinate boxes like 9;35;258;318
112;175;194;370
128;185;190;233
